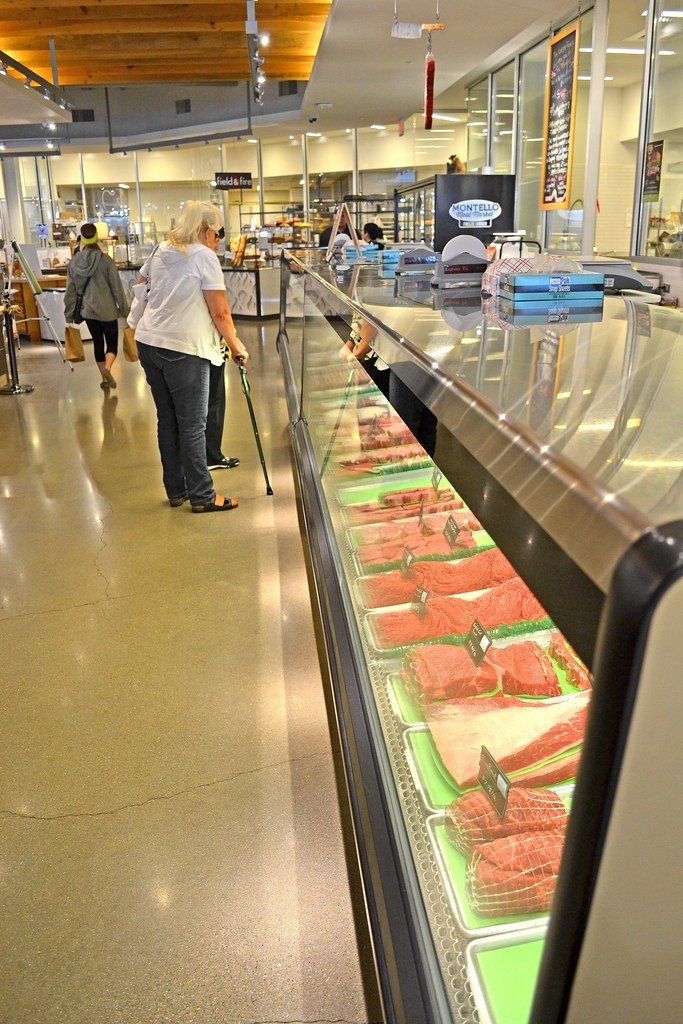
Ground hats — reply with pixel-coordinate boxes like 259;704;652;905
334;205;344;214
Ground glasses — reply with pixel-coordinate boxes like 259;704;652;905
205;224;219;243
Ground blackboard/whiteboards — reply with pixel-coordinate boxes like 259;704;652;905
539;23;579;210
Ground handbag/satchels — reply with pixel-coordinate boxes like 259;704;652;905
123;327;139;362
64;322;85;362
127;244;160;329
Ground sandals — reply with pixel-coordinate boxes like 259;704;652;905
192;494;238;513
169;491;190;506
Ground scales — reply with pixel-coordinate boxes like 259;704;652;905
383;242;432;253
579;256;654;295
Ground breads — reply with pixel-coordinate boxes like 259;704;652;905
265;216;311;243
229;234;247;267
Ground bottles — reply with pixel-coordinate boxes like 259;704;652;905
488;236;529;267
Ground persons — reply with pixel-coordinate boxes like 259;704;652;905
319;209;362;247
63;224;130;388
337;293;438;478
363;223;384;250
43;254;60;268
134;200;249;513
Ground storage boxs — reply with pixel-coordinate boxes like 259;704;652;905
341;252;379;264
480;289;603;331
378;250;410;263
378;264;397;279
482;258;604;301
341;240;378;254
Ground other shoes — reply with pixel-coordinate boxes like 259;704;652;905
101;381;109;389
103;369;117;389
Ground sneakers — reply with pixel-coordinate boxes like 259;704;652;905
207;457;239;470
